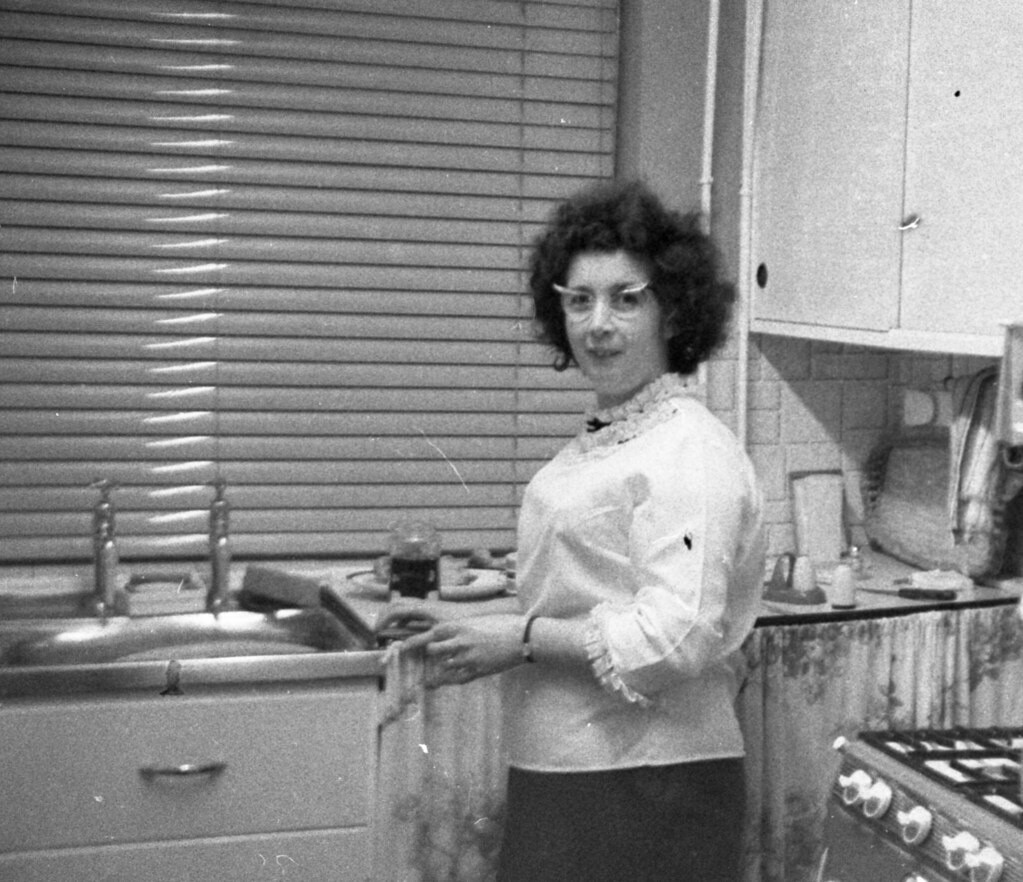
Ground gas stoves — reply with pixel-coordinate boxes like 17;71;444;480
830;725;1023;881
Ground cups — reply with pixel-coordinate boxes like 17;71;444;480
389;519;440;610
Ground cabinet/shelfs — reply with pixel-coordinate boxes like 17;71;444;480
384;600;1023;882
1;664;382;882
633;2;1023;360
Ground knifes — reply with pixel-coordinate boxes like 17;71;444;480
858;588;956;601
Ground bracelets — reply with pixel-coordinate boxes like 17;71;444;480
522;615;540;662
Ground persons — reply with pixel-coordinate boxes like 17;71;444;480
371;170;765;881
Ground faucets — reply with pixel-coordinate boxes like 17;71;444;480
205;479;234;613
84;475;131;618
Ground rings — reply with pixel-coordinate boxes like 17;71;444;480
448;658;457;668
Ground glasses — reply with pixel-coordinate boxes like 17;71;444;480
559;287;672;324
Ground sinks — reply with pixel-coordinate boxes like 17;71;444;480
17;610;357;667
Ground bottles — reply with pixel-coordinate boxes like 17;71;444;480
832;565;855;609
771;553;796;591
791;555;816;596
